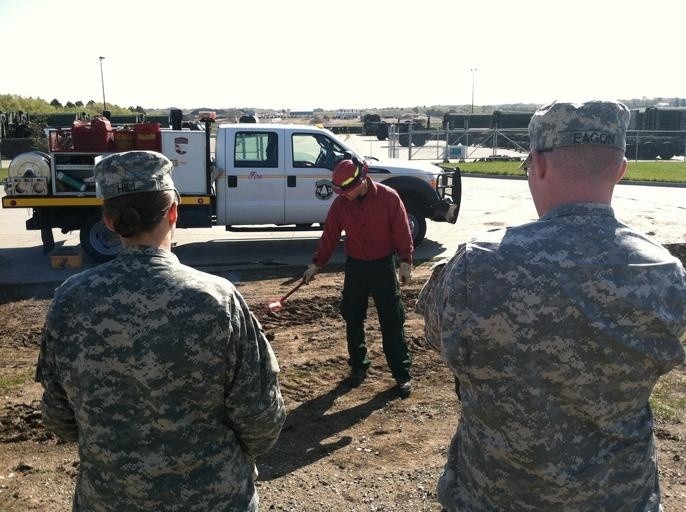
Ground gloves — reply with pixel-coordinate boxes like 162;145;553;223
399;262;412;287
302;263;322;284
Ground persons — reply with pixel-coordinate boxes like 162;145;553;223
36;149;287;512
303;159;414;392
415;101;686;512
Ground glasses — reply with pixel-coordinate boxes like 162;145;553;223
524;149;552;177
331;182;347;190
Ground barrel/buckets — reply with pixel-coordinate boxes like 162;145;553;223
7;151;52;193
112;128;134;151
71;116;113;152
134;121;162;153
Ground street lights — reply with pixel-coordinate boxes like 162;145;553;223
98;54;107;110
470;65;478;113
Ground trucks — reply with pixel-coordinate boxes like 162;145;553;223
237;114;260;137
364;107;686;159
2;120;463;262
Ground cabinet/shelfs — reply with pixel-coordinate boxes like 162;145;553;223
49;151;115;197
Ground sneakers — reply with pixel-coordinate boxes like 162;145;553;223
350;368;366;387
397;378;411;395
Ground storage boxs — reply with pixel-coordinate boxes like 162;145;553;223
50;247;84;268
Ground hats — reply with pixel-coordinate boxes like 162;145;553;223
519;100;631;171
331;159;368;195
93;150;181;205
